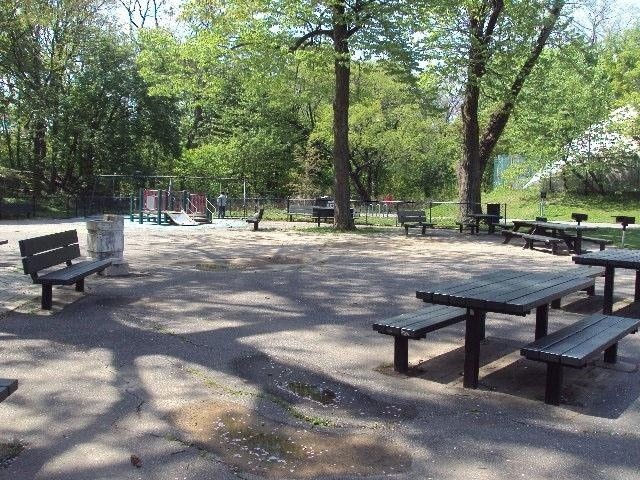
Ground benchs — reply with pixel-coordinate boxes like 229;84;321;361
457;222;475;234
519;314;640;404
549;265;607;308
571;235;614;251
493;222;513;232
246;209;263;230
397;211;435;235
287;206;322;222
373;304;467;372
312;208;354;227
522;235;563;256
502;230;524;249
18;230;111;309
0;379;18;402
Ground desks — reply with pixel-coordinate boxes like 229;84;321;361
463;214;502;235
504;220;536;248
572;248;640;315
529;223;600;255
416;269;594;389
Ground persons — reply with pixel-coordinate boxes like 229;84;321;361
217;191;227;218
